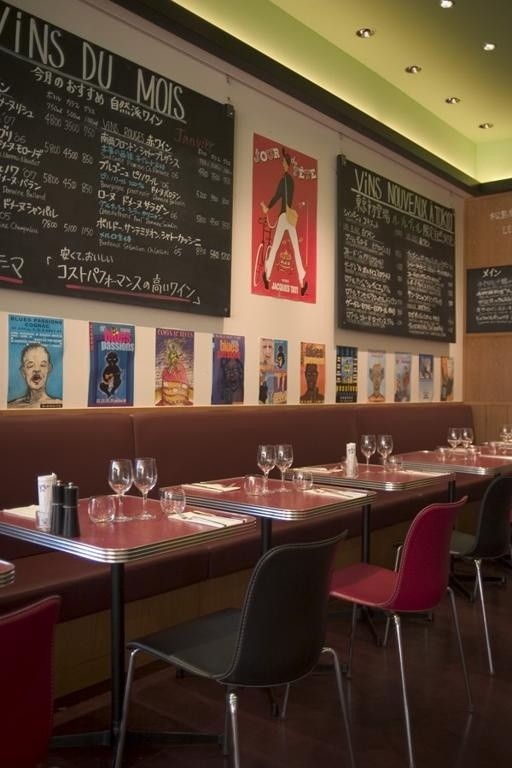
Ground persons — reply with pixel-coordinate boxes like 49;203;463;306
301;363;324;400
98;352;125;398
258;145;309;299
439;357;453;401
261;339;274;364
276;346;286;369
160;340;192;385
367;362;386;401
9;342;63;410
402;364;410;394
216;355;243;404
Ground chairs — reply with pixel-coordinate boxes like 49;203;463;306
113;531;358;768
0;595;65;768
382;473;512;677
281;495;471;767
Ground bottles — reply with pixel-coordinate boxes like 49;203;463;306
62;480;82;539
50;478;64;536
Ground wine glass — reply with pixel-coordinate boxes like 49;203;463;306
445;426;461;456
107;458;136;522
275;444;294;494
134;456;159;524
254;444;276;496
359;433;375;475
498;423;511;453
461;426;474;460
376;435;393;474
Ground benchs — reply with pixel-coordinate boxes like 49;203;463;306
1;401;487;701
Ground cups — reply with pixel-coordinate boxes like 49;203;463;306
385;455;403;473
35;511;50;534
241;473;270;499
341;457;360;479
467;444;481;462
88;496;115;525
484;440;500;455
292;468;313;492
161;488;187;514
434;446;449;463
